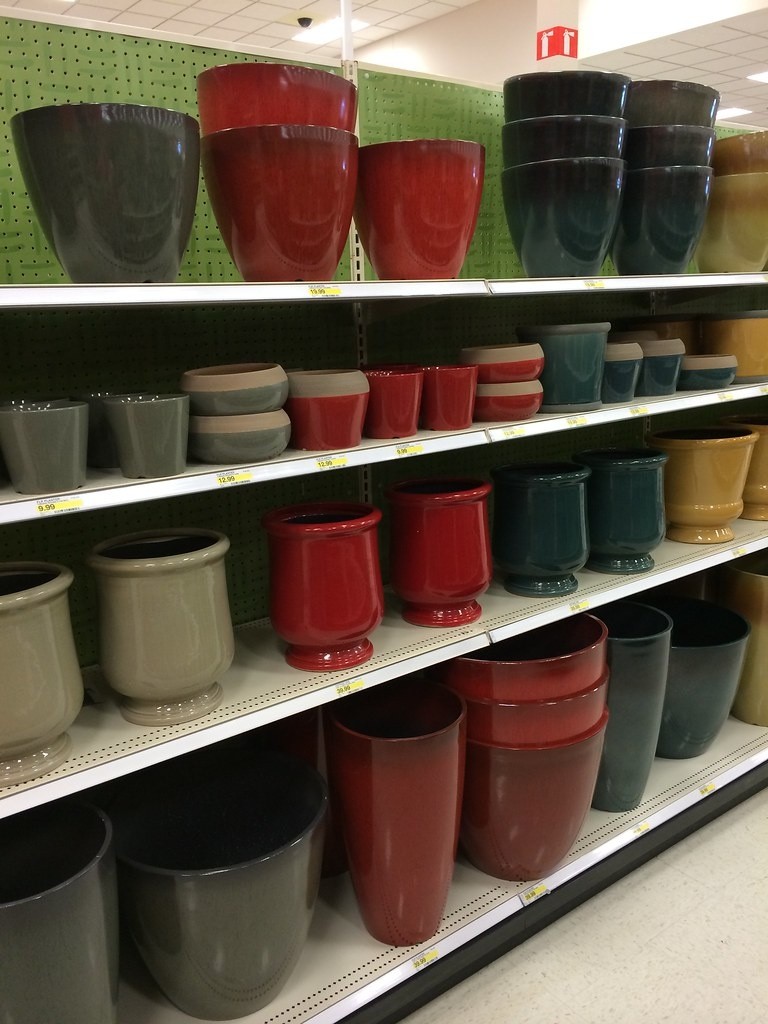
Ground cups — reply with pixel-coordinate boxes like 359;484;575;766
0;395;89;495
88;524;238;727
387;473;494;629
89;388;189;479
1;559;84;790
462;343;544;422
694;130;768;275
353;138;486;281
609;80;721;276
284;369;371;452
702;549;768;727
264;500;386;673
515;308;768;415
591;602;673;811
499;69;631;277
357;361;478;441
198;62;359;284
180;363;292;466
626;597;752;759
324;679;470;947
572;447;671;576
0;801;124;1024
433;612;610;883
644;410;768;546
101;743;329;1021
8;103;201;282
490;460;592;598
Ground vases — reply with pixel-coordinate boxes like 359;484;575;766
0;62;768;1024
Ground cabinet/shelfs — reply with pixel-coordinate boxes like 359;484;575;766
0;6;768;1024
